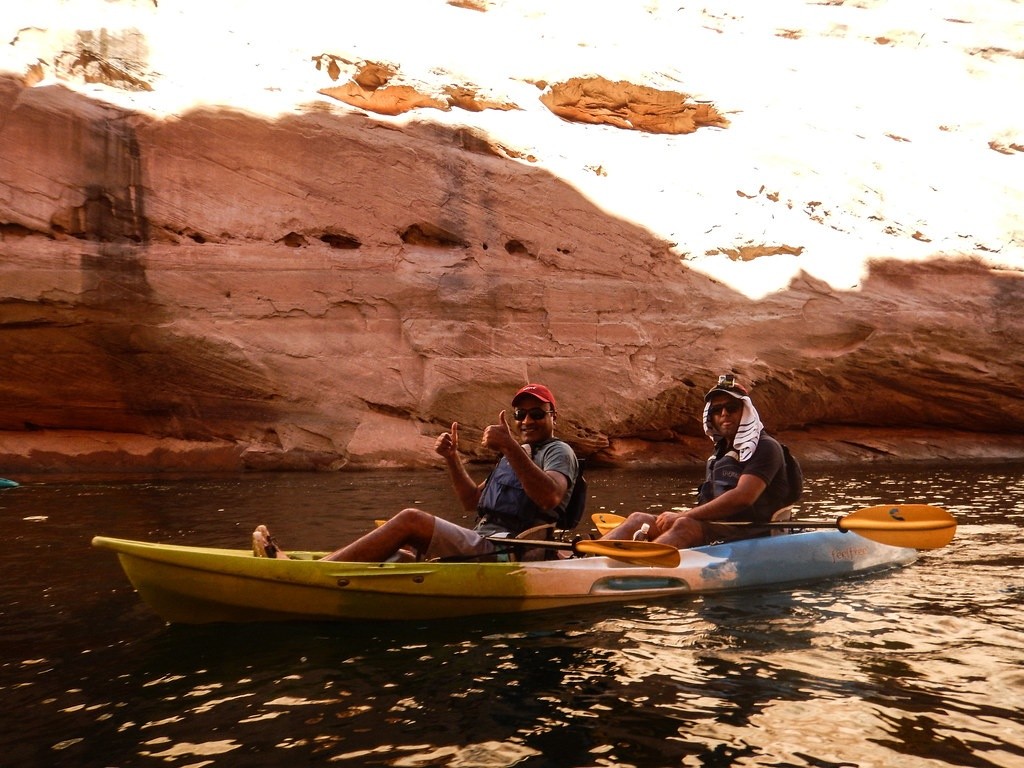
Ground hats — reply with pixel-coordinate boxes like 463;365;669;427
703;373;751;402
512;384;557;408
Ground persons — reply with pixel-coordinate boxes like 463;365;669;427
251;382;579;574
592;378;785;552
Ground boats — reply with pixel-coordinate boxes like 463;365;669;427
93;530;918;622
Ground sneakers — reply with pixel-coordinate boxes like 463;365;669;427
252;525;281;558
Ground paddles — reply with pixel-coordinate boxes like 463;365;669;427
374;517;683;570
591;503;958;553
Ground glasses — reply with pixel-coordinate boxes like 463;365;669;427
708;401;739;413
513;407;555;420
